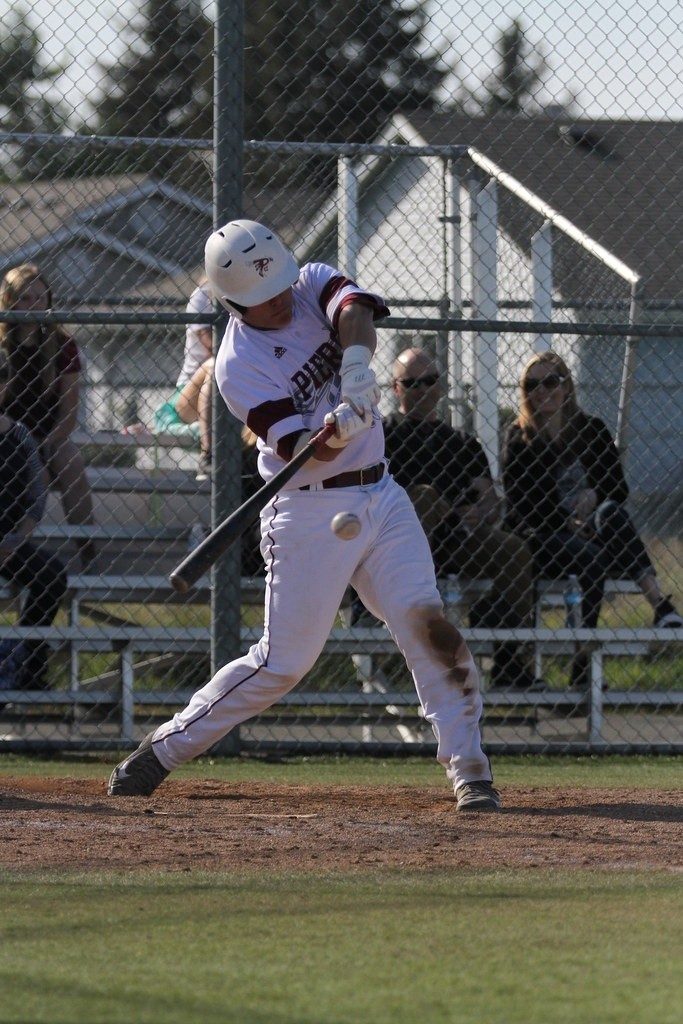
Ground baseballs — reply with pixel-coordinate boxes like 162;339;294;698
331;511;362;541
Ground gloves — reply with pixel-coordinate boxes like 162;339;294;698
339;346;382;415
323;395;372;449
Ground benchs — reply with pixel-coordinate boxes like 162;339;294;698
25;432;682;738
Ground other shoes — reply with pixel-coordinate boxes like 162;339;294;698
568;674;609;693
654;594;683;629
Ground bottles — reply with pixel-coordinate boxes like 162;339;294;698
189;523;208;590
564;575;582;629
445;575;461;628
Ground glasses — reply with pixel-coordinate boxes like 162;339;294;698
524;374;567;392
397;373;439;389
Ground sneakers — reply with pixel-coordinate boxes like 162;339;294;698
107;730;171;797
456;781;503;811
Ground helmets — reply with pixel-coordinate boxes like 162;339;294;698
205;220;300;321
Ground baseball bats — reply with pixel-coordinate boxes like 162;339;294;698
168;423;335;593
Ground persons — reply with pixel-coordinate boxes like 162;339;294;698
499;351;683;691
0;264;101;574
351;346;546;693
0;349;68;690
107;217;501;811
153;289;258;482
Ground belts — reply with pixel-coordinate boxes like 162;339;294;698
299;462;385;492
